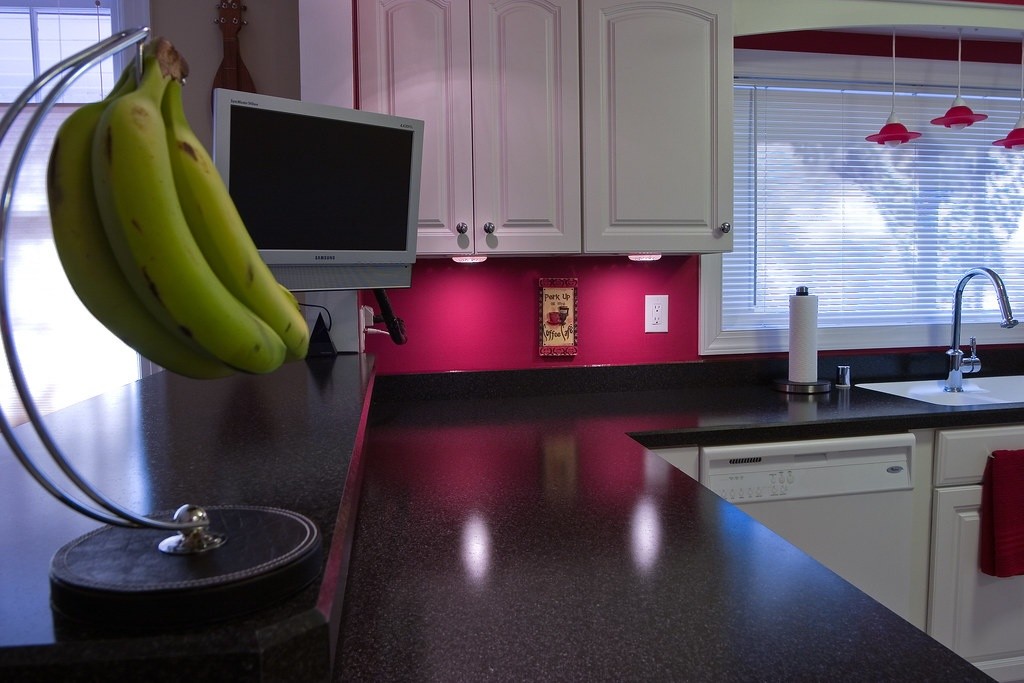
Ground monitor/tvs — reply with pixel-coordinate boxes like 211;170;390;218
213;87;424;292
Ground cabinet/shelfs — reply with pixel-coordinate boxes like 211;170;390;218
580;0;734;256
356;0;583;258
925;425;1024;683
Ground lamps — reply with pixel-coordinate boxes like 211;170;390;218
991;37;1024;152
930;29;988;132
865;27;922;148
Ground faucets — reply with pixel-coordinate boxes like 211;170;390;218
944;267;1019;392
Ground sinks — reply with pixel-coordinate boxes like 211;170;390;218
854;375;1024;407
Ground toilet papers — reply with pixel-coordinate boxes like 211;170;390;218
788;294;818;383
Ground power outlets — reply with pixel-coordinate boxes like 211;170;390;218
644;294;669;333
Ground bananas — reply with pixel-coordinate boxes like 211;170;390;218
47;38;309;377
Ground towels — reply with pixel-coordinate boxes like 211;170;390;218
978;448;1024;577
